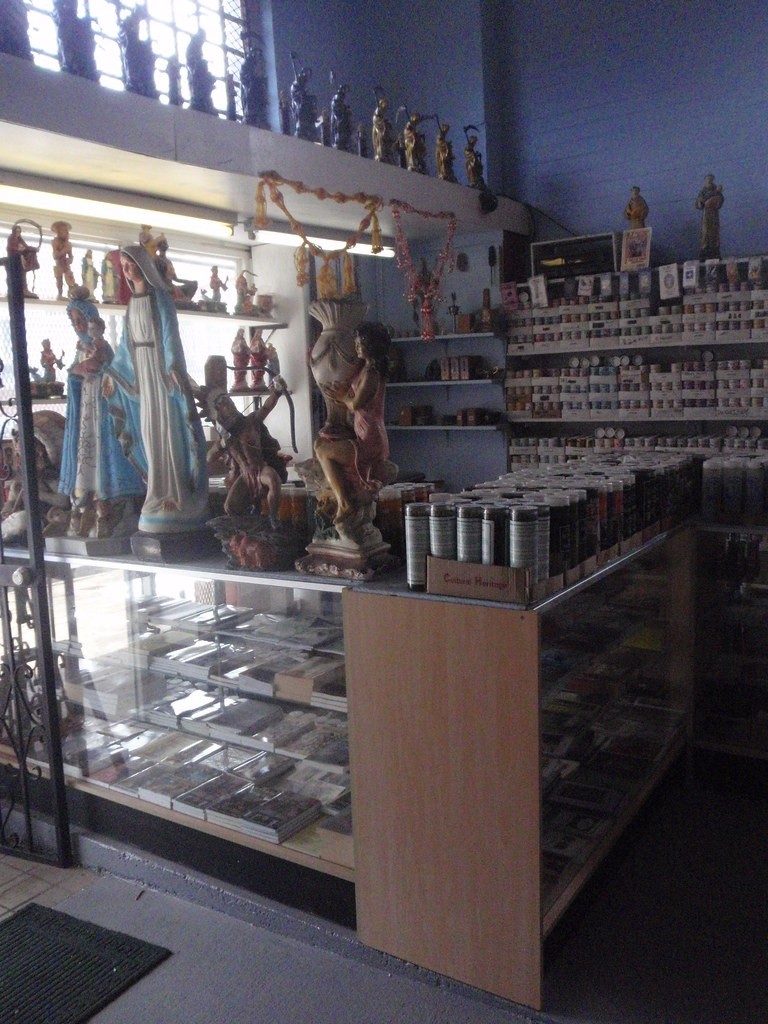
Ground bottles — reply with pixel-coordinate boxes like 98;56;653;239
406;454;768;593
257;481;435;557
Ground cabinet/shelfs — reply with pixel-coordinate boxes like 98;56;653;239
341;516;703;1010
0;539;405;929
507;337;768;422
384;332;510;431
697;524;768;762
0;296;293;404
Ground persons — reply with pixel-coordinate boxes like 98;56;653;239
314;320;391;524
623;185;650;230
695;174;724;260
63;303;118;536
121;246;208;517
229;325;288;394
35;336;64;388
7;220;227;312
206;377;284;531
241;33;486;190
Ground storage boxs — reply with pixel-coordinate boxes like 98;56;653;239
457;408;487;426
399;406;414;425
456;314;475;333
440;357;476;380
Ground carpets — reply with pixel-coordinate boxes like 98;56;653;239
0;903;173;1024
